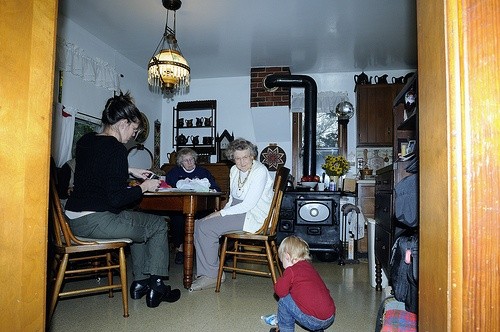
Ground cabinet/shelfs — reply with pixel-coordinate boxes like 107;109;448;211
390;72;421;160
373;161;411;290
357;183;376;252
354;83;395;146
172;100;216;156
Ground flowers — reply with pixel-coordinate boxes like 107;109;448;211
321;155;351;178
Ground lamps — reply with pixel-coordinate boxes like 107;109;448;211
148;0;190;95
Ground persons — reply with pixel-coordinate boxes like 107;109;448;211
189;138;275;291
270;236;336;332
166;148;221;265
63;89;181;308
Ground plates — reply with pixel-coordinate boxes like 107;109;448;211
127;186;163;189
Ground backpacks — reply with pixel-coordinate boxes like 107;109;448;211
389;235;418;315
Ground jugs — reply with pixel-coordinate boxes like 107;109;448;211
391;76;403;84
190;135;199;145
205;116;212;127
374;74;388;84
195;117;204;127
185;119;193;127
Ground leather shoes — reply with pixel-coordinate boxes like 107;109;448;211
146;289;181;308
129;281;171;300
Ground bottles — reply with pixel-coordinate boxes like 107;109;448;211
329;180;336;192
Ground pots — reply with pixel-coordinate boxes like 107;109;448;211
360;168;373;175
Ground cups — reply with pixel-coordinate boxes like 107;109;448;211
177;117;184;128
203;137;213;145
318;183;324;191
210;155;217;163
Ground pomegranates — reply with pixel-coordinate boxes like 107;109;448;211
301;175;320;182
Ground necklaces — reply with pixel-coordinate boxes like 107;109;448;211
238;163;253;190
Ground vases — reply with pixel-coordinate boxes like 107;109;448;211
330;175;340;191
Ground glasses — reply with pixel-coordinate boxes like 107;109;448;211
232;155;251;161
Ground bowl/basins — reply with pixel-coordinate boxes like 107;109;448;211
301;182;318;191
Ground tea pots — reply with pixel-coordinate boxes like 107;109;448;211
354;72;372;84
166;150;176;165
405;91;415;104
175;134;190;145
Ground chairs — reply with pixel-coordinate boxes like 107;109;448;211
214;166;290;293
49;154;133;318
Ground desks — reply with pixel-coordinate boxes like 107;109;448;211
92;191;227;289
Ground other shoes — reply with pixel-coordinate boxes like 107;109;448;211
270;327;281;332
190;271;225;291
